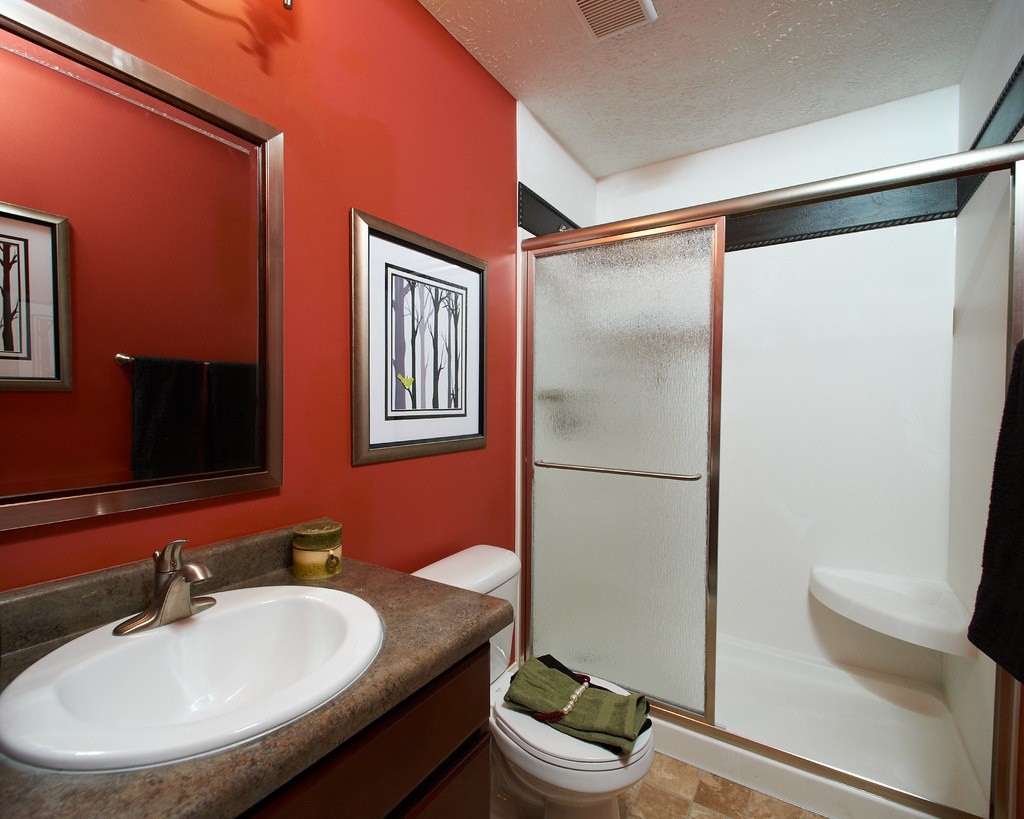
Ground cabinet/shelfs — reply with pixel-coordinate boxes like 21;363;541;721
0;527;514;819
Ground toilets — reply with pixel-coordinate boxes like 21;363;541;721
409;543;654;818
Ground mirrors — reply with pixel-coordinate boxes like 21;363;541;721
0;0;286;547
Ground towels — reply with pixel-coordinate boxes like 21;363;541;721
503;653;652;756
967;339;1024;683
205;361;263;471
130;354;204;478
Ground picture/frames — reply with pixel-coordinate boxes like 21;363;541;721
0;203;78;392
348;208;489;467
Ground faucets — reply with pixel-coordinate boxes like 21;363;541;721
112;537;218;637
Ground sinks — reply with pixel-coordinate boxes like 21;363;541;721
1;585;385;771
